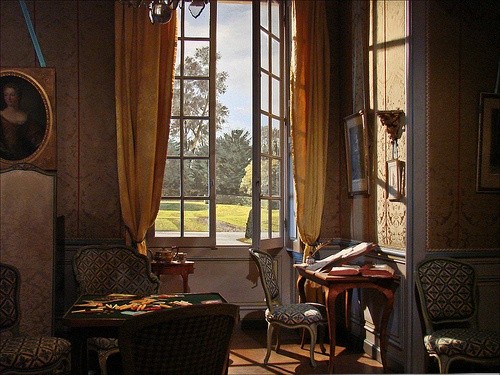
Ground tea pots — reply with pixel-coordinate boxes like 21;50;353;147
147;246;179;263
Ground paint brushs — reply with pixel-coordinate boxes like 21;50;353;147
312;241;331;256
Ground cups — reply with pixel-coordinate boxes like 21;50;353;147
175;252;187;263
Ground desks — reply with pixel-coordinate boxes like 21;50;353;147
152;260;194;291
293;264;397;375
61;292;230;375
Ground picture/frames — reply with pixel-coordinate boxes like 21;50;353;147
385;160;402;201
475;92;500;194
0;70;53;166
342;110;370;197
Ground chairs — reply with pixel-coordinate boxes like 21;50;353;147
0;263;71;375
415;258;500;375
249;248;328;369
72;245;161;297
